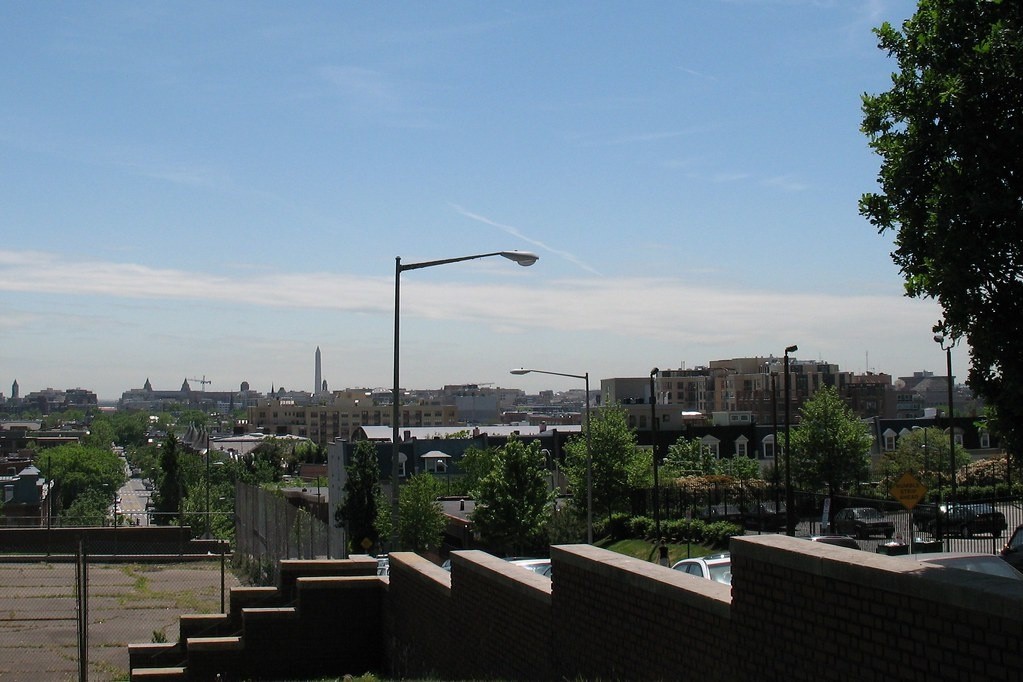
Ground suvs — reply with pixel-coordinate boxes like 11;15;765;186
793;534;861;550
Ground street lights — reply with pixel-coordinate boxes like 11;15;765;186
390;252;539;553
283;475;321;520
784;346;797;536
206;434;218;539
770;371;779;478
509;369;593;547
921;445;942;462
934;329;963;504
102;484;117;556
650;367;661;538
541;449;556;512
912;426;928;470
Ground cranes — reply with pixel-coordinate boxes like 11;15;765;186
187;375;211;391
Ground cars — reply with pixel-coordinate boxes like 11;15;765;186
132;469;142;478
744;501;799;531
670;552;731;586
913;502;961;532
114;497;121;503
826;508;895;540
1001;524;1023;573
112;506;121;514
927;504;1008;539
696;504;744;530
